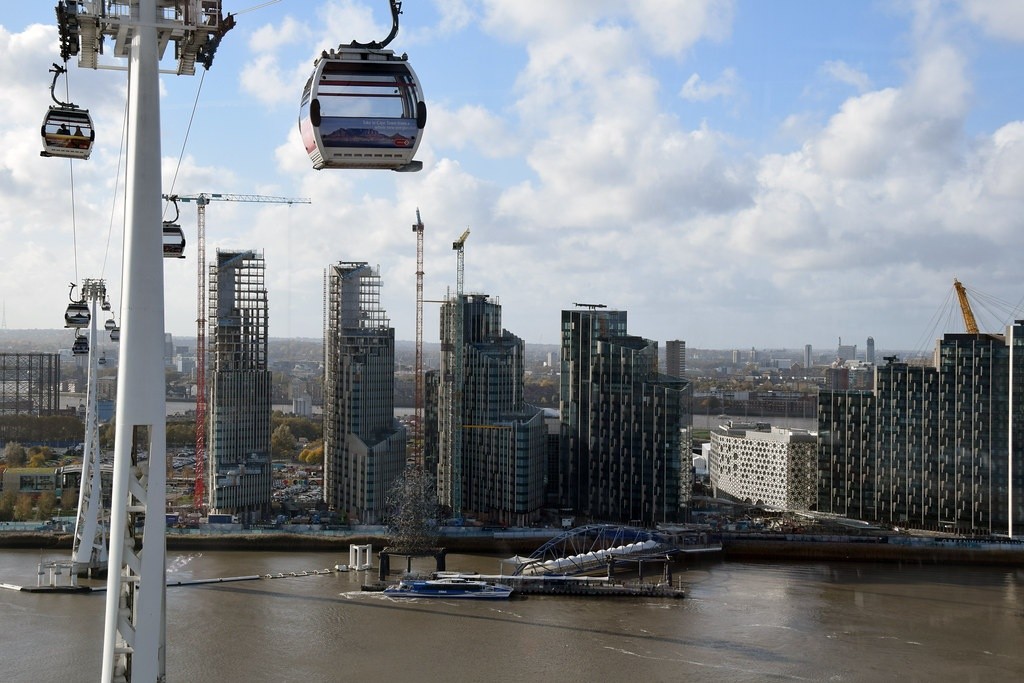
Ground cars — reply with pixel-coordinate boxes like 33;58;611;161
137;448;148;461
272;463;324;504
170;446;208;469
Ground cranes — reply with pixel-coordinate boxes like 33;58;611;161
162;193;313;515
413;208;424;472
452;228;470;517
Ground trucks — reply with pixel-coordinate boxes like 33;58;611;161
75;443;85;451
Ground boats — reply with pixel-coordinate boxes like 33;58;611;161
384;573;513;601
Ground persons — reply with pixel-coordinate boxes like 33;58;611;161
56;124;71;135
74;126;84;136
